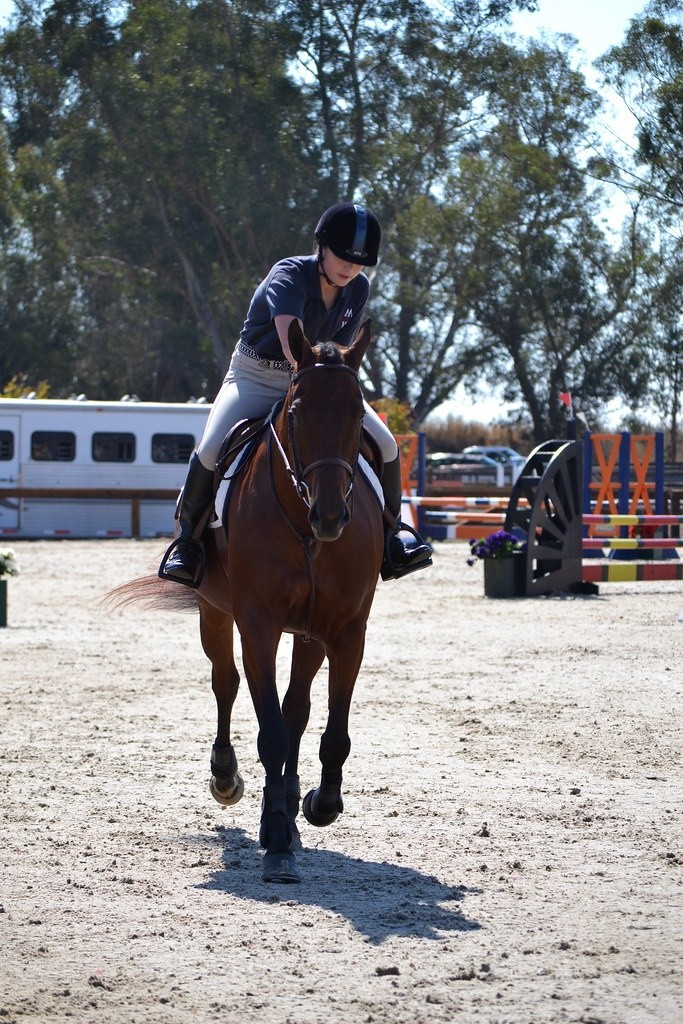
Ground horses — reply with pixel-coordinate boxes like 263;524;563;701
95;318;383;883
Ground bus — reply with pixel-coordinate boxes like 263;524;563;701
0;391;214;540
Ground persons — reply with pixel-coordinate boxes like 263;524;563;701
164;204;434;582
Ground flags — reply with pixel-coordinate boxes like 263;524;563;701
560;393;571;407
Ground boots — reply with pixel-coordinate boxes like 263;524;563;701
381;447;433;581
165;448;227;580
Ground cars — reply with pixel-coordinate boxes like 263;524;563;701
464;445;523;474
425;453;500;481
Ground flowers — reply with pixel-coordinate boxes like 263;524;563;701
0;547;21;577
467;530;519;567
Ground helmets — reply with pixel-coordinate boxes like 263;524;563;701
314;202;381;266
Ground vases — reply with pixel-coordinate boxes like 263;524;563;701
0;581;8;627
484;556;518;598
